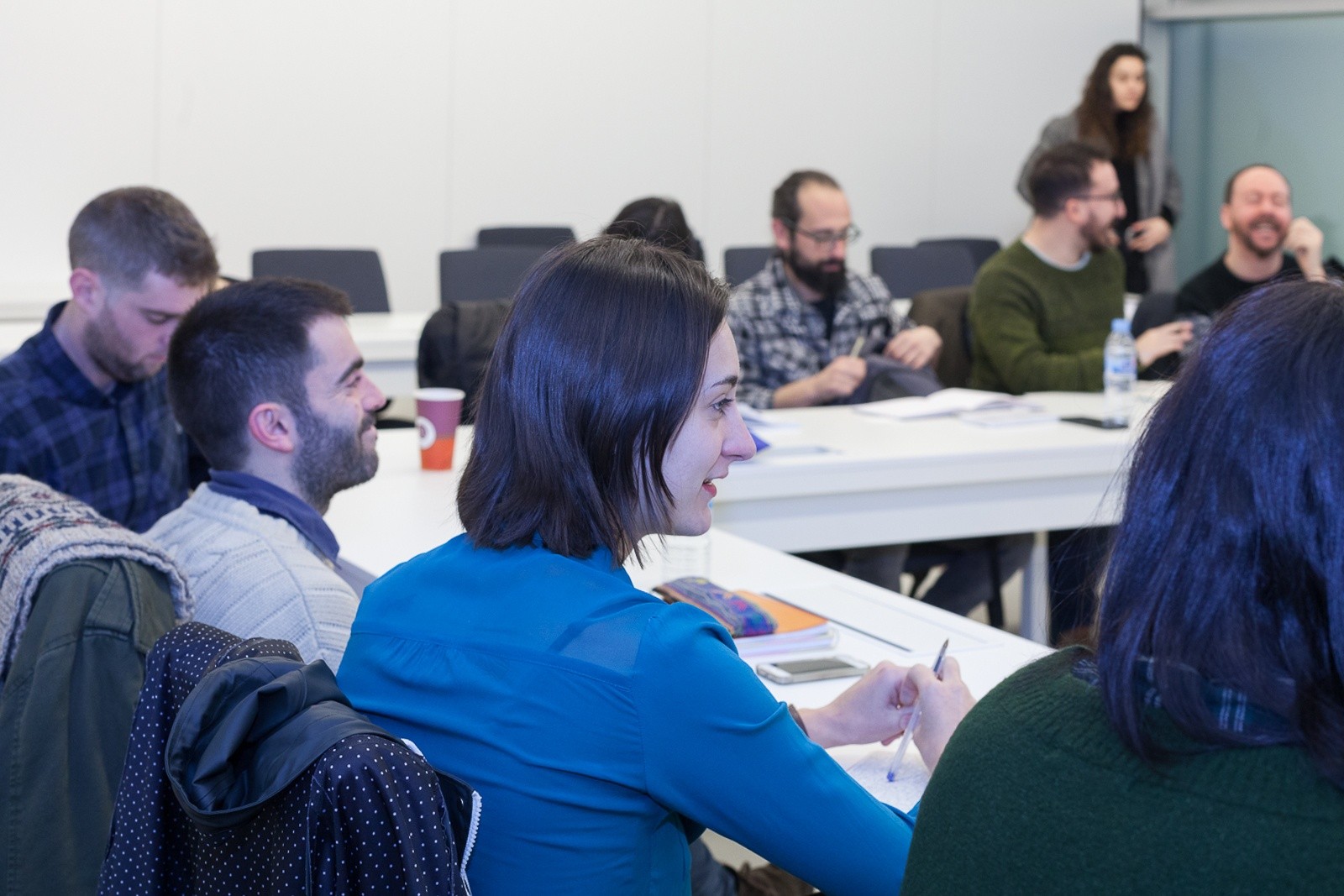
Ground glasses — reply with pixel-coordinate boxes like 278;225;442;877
781;218;860;250
1060;189;1123;207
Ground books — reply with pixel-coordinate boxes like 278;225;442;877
652;576;836;655
856;388;1020;418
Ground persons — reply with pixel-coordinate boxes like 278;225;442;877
1017;42;1182;295
335;237;975;896
902;277;1343;896
0;185;815;895
722;142;1344;645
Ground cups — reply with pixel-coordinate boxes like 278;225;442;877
413;387;465;470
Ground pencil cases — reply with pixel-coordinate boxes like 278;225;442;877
650;575;779;638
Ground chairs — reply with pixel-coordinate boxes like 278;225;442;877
253;225;1176;425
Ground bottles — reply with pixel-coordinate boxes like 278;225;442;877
1102;318;1136;428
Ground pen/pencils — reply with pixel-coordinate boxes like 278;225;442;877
886;637;952;784
851;335;865;358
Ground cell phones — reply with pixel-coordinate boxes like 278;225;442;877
757;653;871;684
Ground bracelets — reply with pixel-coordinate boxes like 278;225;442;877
789;704;810;738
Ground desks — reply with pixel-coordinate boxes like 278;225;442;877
323;383;1173;894
